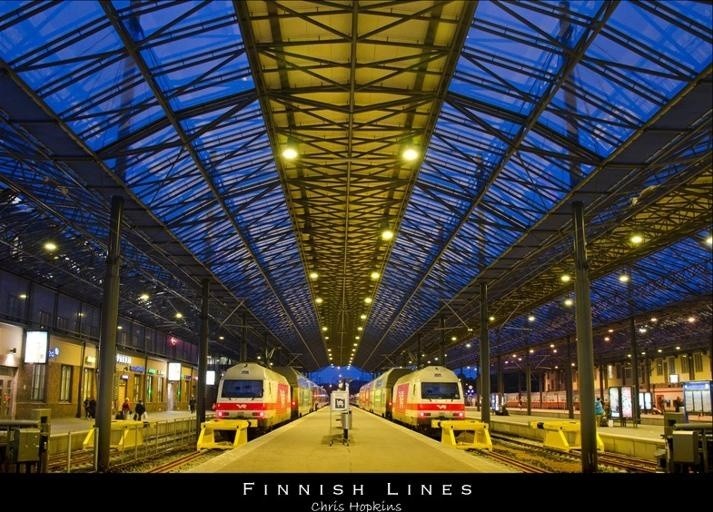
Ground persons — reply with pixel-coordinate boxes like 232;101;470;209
675;396;682;412
83;397;96;421
122;397;144;421
189;395;196;414
658;397;664;415
595;397;612;424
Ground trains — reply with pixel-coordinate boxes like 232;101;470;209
349;366;465;436
216;363;330;436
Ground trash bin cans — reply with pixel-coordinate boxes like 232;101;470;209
31;409;52;435
341;410;352;429
663;411;686;439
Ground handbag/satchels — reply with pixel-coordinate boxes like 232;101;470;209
115;411;126;420
143;412;148;421
133;413;137;420
